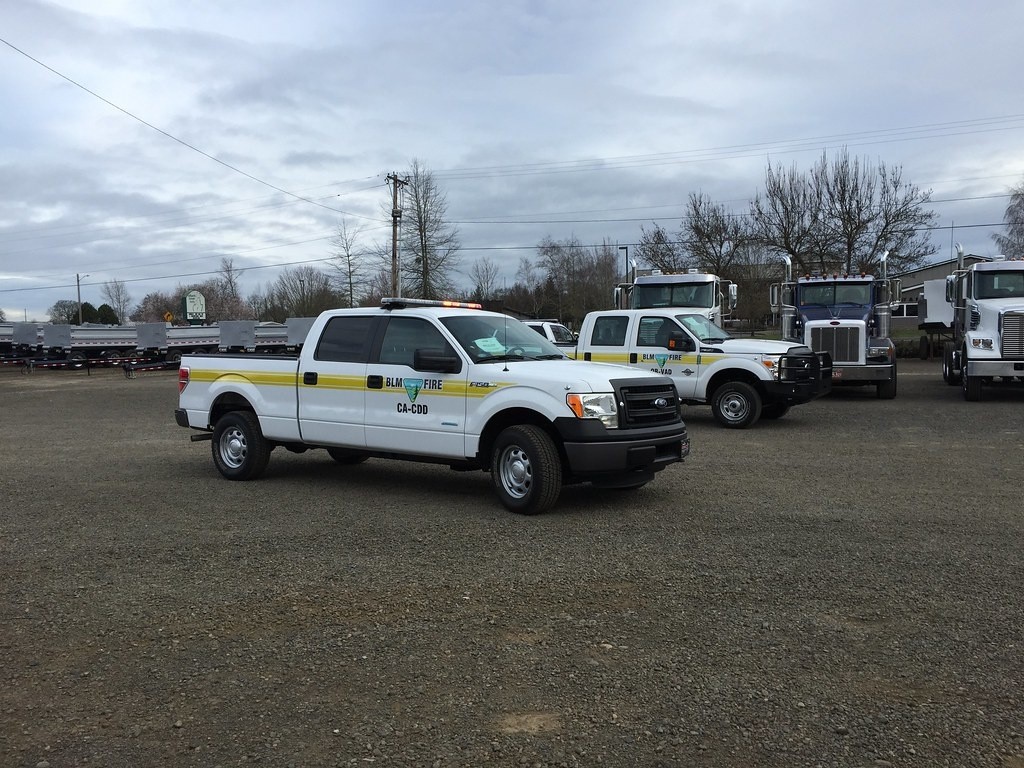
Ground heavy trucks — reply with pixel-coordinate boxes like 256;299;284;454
614;258;738;344
769;253;903;399
941;242;1024;400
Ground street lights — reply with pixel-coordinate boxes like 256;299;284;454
300;280;305;317
619;246;628;284
77;274;90;324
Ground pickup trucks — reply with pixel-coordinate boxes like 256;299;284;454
174;298;692;516
484;310;834;431
491;321;579;346
